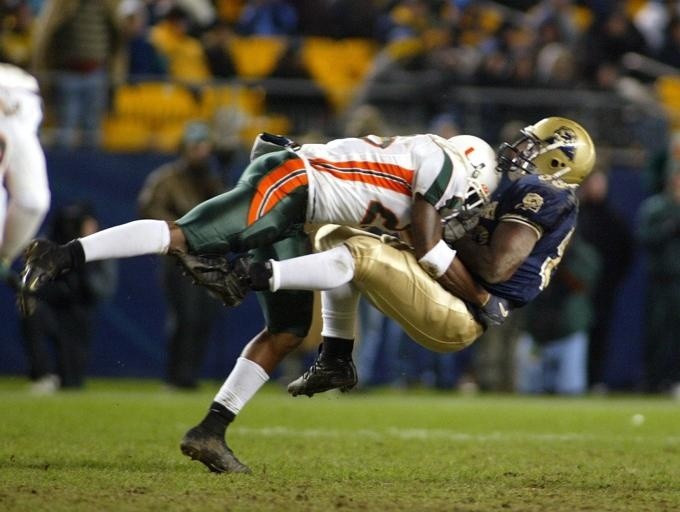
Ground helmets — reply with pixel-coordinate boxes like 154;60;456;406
443;134;502;228
497;115;597;191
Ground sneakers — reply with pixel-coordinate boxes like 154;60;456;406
174;249;256;308
288;342;358;395
18;239;72;317
178;426;253;474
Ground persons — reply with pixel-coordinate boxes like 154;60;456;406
631;160;679;395
574;167;633;400
510;234;602;396
165;115;598;398
0;60;53;279
22;185;106;401
0;1;679;160
134;121;229;389
16;114;502;480
